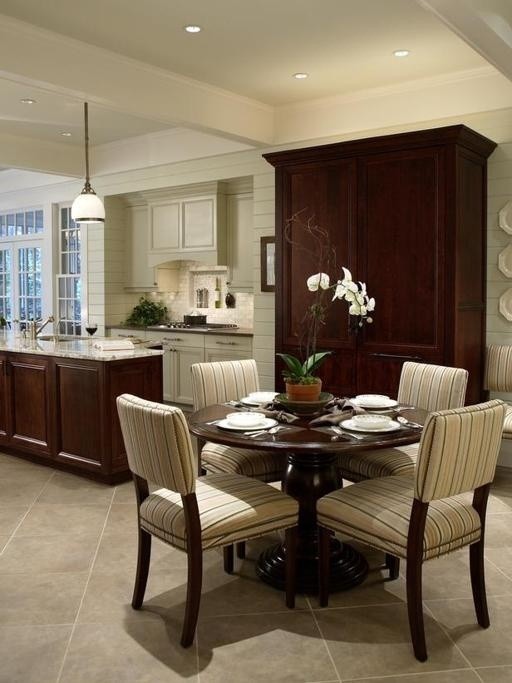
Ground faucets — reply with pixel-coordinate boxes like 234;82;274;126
33;316;54;340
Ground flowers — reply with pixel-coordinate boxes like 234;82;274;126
274;207;376;384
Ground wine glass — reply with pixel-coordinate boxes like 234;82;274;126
86;323;97;342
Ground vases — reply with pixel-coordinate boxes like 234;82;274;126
285;378;322;401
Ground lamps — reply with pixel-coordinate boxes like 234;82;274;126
70;102;105;224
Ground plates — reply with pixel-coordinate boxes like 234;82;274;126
216;419;283;430
226;412;265;426
240;396;286;405
340;419;403;432
350;413;395;429
357;392;391;404
249;391;281;403
349;397;398;407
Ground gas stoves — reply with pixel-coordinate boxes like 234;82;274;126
156;319;236;331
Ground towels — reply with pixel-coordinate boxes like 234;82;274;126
93;340;135;350
332;398;355;410
265;401;282;409
266;411;297;422
311;411;352;427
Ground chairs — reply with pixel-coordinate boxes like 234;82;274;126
315;399;508;663
189;358;290;559
115;392;300;649
334;361;469;580
483;343;512;439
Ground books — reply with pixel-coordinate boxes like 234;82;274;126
94;340;136;351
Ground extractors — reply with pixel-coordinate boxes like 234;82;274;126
146;252;226;273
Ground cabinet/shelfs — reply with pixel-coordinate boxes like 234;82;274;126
206;333;253;361
260;122;498;407
146;331;204;407
106;328;146;343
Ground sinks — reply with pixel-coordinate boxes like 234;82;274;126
39;335;89;342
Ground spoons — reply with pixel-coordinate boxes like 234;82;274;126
243;426;283;438
397;416;424;429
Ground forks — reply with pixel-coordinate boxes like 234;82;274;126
330;424;364;441
